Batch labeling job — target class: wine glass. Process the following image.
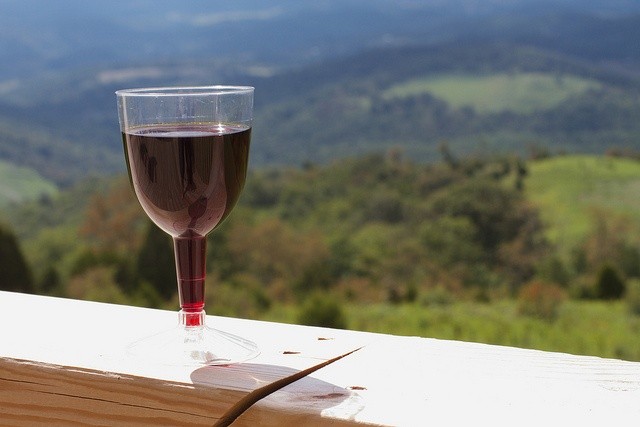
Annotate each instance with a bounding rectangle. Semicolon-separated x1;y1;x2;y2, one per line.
116;90;254;362
